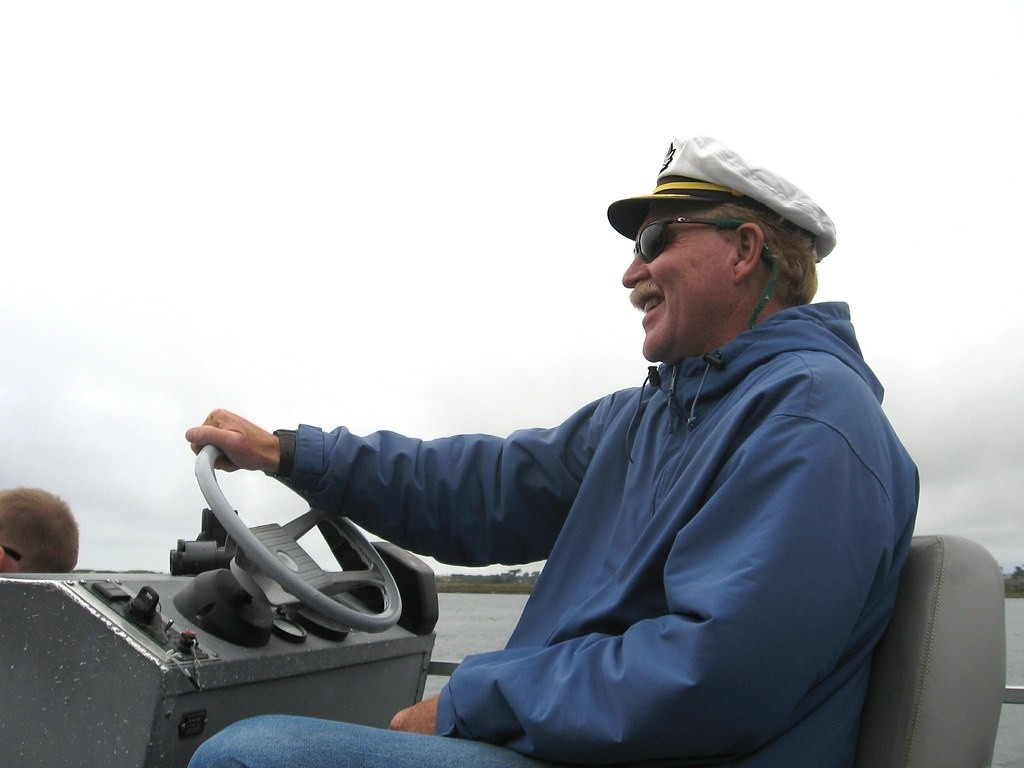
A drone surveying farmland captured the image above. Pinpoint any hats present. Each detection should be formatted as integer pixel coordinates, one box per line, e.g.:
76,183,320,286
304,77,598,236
607,135,837,263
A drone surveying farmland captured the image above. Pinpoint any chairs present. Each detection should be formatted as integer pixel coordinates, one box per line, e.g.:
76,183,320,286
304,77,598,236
854,535,1007,768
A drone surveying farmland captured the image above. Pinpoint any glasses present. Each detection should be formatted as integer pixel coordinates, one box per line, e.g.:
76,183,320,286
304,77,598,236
633,216,772,264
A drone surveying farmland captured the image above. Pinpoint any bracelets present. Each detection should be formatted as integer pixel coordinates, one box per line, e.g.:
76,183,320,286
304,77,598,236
273,429,296,479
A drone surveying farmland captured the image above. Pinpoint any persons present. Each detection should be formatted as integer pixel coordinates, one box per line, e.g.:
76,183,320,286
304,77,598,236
0,486,79,573
186,135,921,767
